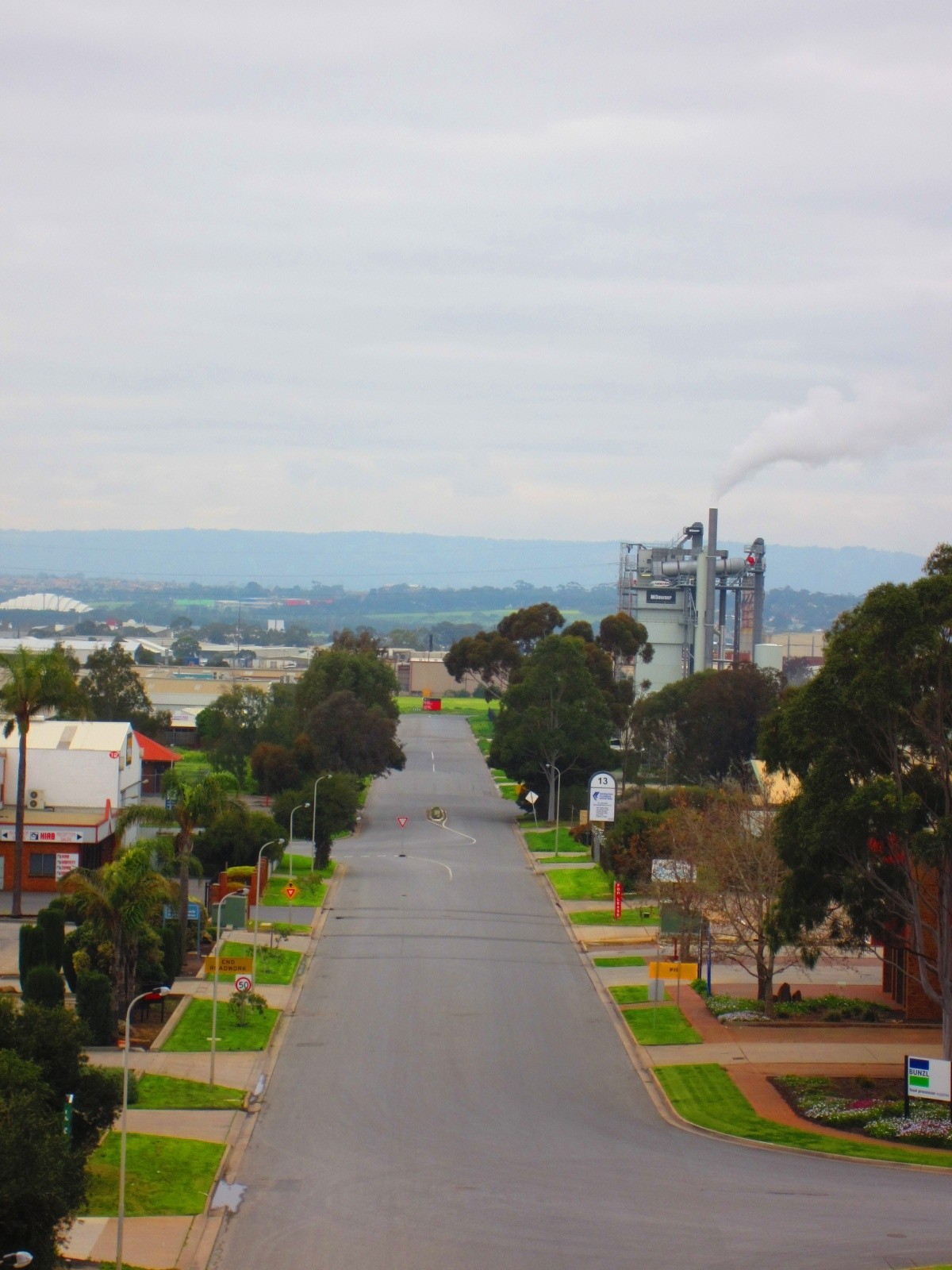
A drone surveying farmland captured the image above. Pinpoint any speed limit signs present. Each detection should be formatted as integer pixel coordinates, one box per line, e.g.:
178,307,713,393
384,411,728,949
235,977,251,992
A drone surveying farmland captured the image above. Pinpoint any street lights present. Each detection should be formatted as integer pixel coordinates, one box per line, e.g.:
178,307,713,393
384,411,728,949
288,802,310,924
208,888,251,1086
311,774,334,874
252,839,286,997
115,986,173,1269
546,764,559,858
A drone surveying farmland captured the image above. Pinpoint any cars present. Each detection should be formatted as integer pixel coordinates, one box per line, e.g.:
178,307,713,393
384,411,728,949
610,738,635,751
